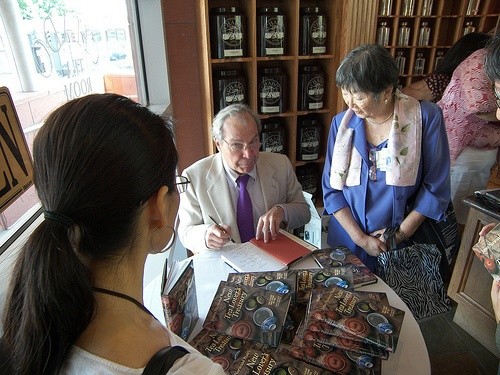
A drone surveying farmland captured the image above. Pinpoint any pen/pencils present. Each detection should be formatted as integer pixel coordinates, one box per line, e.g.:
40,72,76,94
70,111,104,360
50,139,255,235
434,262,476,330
208,215,236,244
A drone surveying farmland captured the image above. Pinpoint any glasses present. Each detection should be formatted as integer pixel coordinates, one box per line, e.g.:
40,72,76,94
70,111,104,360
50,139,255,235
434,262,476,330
222,138,262,151
172,175,191,194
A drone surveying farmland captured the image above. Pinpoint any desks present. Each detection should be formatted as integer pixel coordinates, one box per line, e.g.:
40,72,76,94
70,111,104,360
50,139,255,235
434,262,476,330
143,245,431,375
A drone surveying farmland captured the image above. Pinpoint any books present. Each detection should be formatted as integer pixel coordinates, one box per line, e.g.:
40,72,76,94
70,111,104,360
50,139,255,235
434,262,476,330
471,221,500,284
159,225,406,375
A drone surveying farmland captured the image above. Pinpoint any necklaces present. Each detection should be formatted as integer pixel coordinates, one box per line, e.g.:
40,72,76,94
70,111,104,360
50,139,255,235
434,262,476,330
93,287,159,322
366,111,394,125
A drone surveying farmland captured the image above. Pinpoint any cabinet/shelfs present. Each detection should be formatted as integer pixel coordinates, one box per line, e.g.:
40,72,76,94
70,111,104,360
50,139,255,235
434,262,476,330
197,0,500,211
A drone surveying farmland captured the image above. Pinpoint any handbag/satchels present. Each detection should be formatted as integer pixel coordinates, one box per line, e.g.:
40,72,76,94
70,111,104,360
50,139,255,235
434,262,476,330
375,224,452,321
404,191,462,281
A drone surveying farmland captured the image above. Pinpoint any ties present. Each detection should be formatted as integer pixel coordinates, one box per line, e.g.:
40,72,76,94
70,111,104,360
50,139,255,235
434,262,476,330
236,174,256,244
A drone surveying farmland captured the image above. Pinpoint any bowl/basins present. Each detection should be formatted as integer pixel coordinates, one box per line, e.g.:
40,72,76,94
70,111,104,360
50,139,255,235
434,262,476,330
167,245,397,374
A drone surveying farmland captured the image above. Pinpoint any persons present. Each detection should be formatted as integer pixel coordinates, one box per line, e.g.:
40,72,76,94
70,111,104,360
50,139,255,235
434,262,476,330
321,44,460,277
0,92,225,375
174,104,311,254
401,31,500,357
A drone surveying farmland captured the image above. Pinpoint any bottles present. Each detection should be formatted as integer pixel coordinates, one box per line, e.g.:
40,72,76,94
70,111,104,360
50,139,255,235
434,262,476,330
376,0,481,90
209,6,328,204
